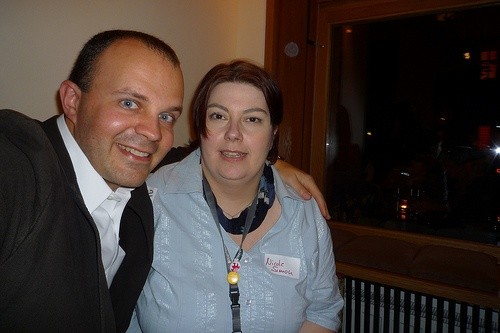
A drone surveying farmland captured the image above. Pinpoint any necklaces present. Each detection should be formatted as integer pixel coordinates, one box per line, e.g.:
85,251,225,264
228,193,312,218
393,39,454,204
200,155,276,236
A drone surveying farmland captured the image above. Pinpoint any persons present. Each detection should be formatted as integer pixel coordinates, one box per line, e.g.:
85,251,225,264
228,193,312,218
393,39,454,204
116,61,345,333
0,30,330,333
